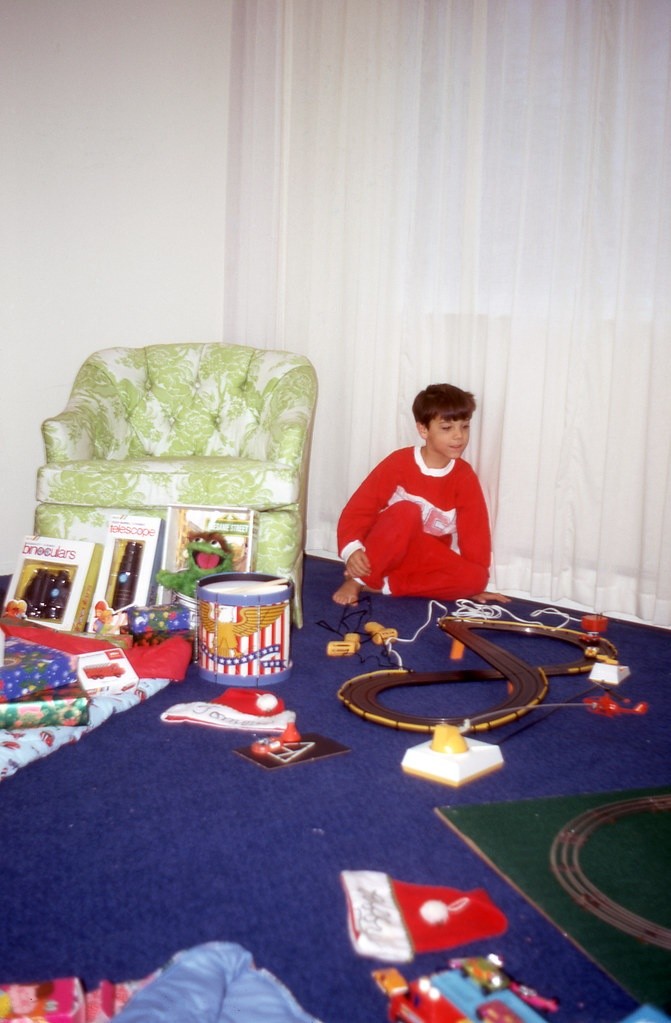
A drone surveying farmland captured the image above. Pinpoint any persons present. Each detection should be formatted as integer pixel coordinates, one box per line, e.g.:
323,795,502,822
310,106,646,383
331,384,512,606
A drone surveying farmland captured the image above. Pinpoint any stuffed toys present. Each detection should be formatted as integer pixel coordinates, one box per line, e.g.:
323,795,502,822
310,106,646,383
156,532,235,599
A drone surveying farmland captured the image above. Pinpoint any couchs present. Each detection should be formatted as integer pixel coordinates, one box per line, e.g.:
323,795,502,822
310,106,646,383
36,337,318,628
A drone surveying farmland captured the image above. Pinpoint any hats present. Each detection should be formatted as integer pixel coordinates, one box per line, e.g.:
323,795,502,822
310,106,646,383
341,869,508,963
161,688,296,733
